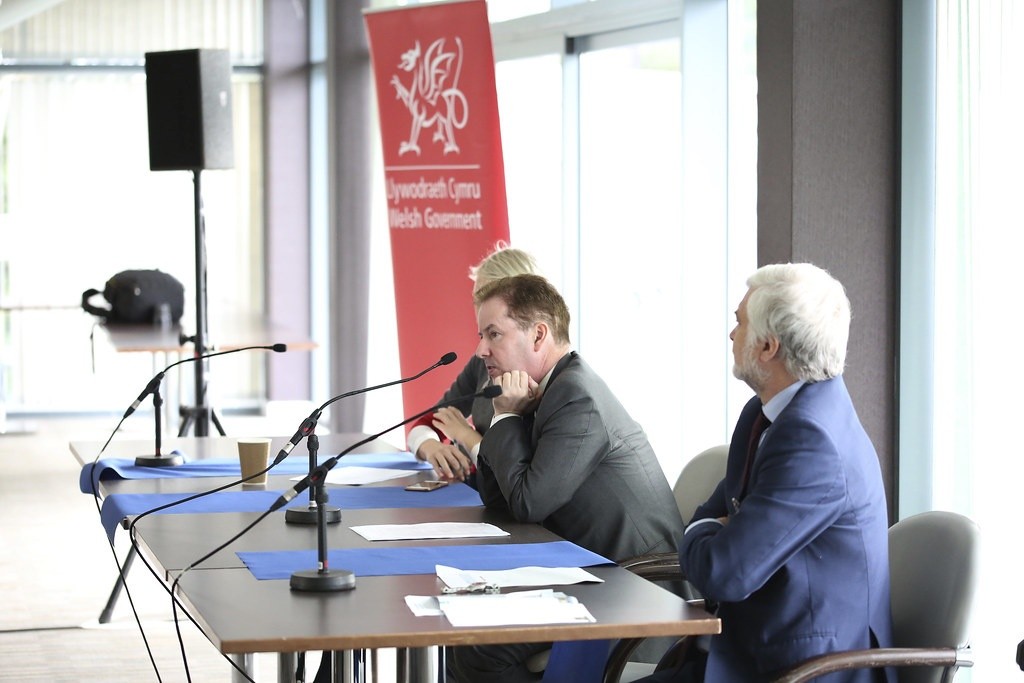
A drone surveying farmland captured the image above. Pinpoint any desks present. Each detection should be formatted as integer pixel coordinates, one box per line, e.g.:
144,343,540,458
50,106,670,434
100,318,317,431
71,432,721,683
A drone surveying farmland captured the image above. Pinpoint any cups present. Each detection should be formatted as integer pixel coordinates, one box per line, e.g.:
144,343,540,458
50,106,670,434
237,438,271,485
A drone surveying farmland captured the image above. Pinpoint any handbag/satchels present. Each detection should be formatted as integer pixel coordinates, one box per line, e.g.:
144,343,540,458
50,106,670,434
82,268,185,325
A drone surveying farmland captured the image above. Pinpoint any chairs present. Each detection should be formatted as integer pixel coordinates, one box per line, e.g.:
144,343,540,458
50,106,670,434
528,442,732,673
655,511,988,683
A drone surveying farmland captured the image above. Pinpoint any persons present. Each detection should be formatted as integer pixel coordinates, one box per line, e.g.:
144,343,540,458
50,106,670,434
310,250,542,682
447,274,687,683
678,262,904,683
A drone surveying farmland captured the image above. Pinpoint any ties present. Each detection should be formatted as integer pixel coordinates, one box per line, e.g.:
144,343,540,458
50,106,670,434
738,410,772,502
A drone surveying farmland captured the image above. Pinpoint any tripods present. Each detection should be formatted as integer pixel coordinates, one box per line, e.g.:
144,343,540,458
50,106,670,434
100,171,227,624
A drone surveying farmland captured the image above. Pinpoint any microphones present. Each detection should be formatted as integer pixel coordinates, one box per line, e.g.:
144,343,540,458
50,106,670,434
123,343,287,418
270,384,503,511
274,352,457,464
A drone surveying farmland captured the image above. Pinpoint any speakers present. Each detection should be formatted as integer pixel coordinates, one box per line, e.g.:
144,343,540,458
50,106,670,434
145,48,234,171
